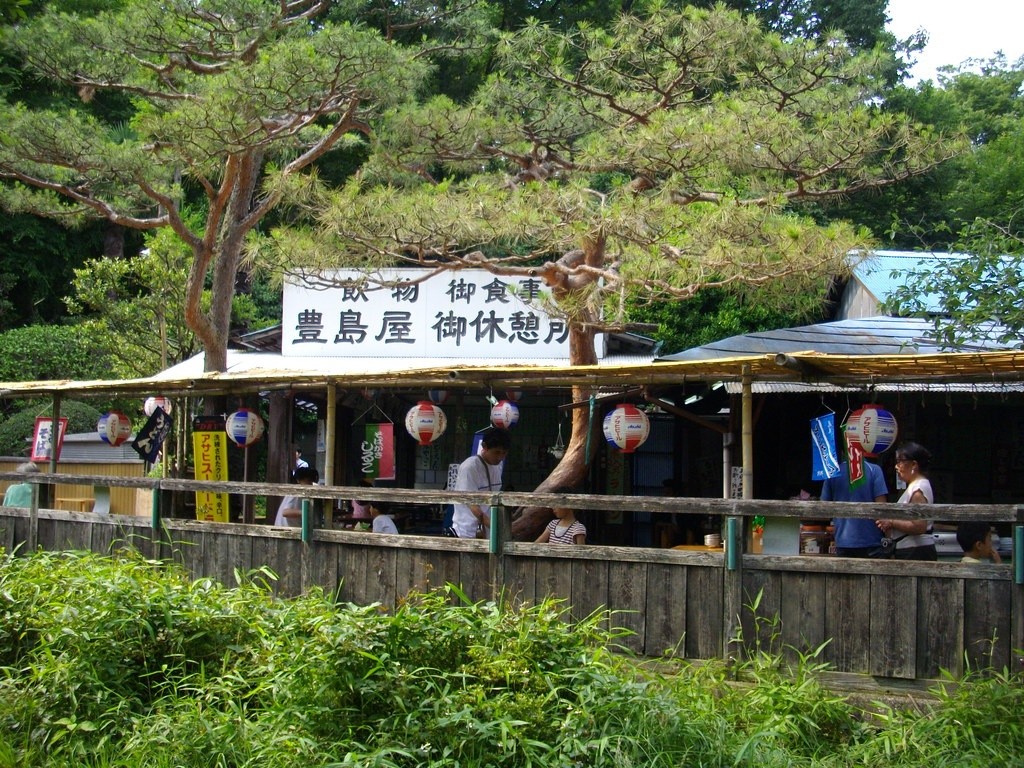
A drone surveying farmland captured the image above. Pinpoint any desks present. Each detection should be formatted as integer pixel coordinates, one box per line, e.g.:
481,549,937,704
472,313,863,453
671,544,723,553
800,531,835,554
336,512,412,534
386,504,429,527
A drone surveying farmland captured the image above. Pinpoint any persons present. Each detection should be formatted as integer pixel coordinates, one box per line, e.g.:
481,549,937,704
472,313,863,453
876,442,938,561
654,478,695,548
957,521,1002,563
451,428,509,540
819,444,888,559
3,461,41,508
274,447,399,534
535,487,587,545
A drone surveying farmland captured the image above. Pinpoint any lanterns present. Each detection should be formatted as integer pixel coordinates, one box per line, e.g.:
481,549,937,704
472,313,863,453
603,404,650,454
405,401,447,446
846,404,899,458
505,391,523,402
98,408,132,448
143,396,172,417
427,389,449,406
491,400,520,431
361,388,378,401
225,407,264,447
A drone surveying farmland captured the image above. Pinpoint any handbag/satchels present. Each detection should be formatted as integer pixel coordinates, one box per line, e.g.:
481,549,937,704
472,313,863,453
868,537,896,559
441,504,457,536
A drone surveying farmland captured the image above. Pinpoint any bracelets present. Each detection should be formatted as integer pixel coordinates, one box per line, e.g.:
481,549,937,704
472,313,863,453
889,519,893,529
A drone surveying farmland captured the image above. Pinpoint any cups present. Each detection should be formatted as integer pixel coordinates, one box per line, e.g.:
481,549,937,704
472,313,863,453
704,534,719,548
1000,538,1012,550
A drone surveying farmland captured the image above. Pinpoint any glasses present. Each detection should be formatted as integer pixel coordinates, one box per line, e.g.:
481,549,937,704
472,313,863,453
894,458,908,465
